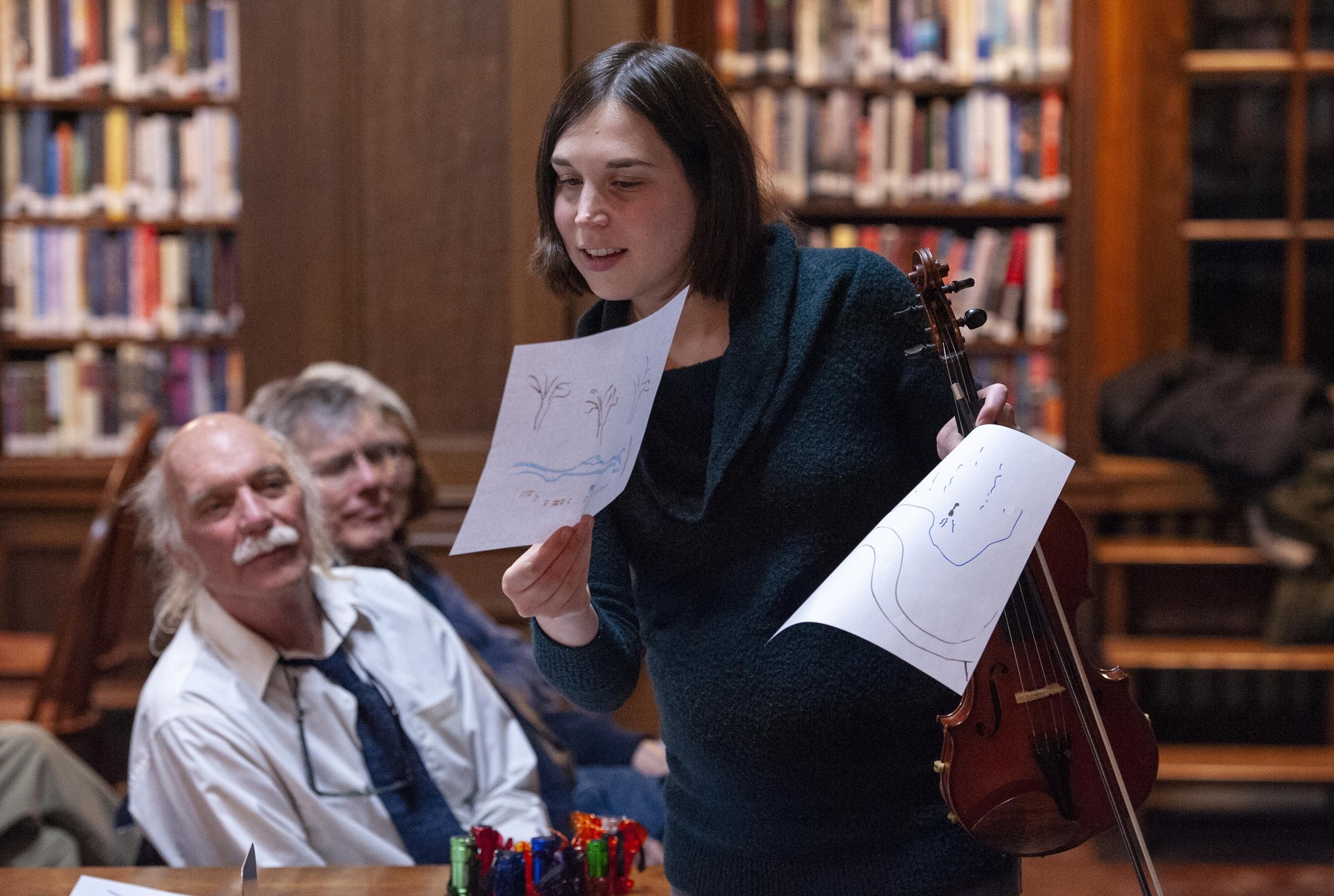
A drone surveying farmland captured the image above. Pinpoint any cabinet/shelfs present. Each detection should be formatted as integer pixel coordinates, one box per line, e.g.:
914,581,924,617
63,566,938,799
1,0,245,625
644,1,1334,846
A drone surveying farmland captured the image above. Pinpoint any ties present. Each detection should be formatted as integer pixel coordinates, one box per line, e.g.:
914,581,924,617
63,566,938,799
277,646,469,865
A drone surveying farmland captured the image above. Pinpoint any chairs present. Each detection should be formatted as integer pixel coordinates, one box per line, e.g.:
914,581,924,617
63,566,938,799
1,412,160,748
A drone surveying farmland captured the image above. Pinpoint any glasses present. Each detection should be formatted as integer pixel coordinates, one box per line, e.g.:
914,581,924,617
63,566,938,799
291,675,417,797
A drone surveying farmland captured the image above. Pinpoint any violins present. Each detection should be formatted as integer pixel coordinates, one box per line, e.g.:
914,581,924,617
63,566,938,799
890,249,1162,861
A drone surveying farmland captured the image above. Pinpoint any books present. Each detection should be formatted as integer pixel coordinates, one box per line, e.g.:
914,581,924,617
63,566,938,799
712,0,1070,455
0,0,246,460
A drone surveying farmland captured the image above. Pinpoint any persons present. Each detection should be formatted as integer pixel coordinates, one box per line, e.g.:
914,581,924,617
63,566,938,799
500,39,1027,896
0,364,672,866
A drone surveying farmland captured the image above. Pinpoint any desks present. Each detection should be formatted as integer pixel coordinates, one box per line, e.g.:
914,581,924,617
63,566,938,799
0,855,670,896
